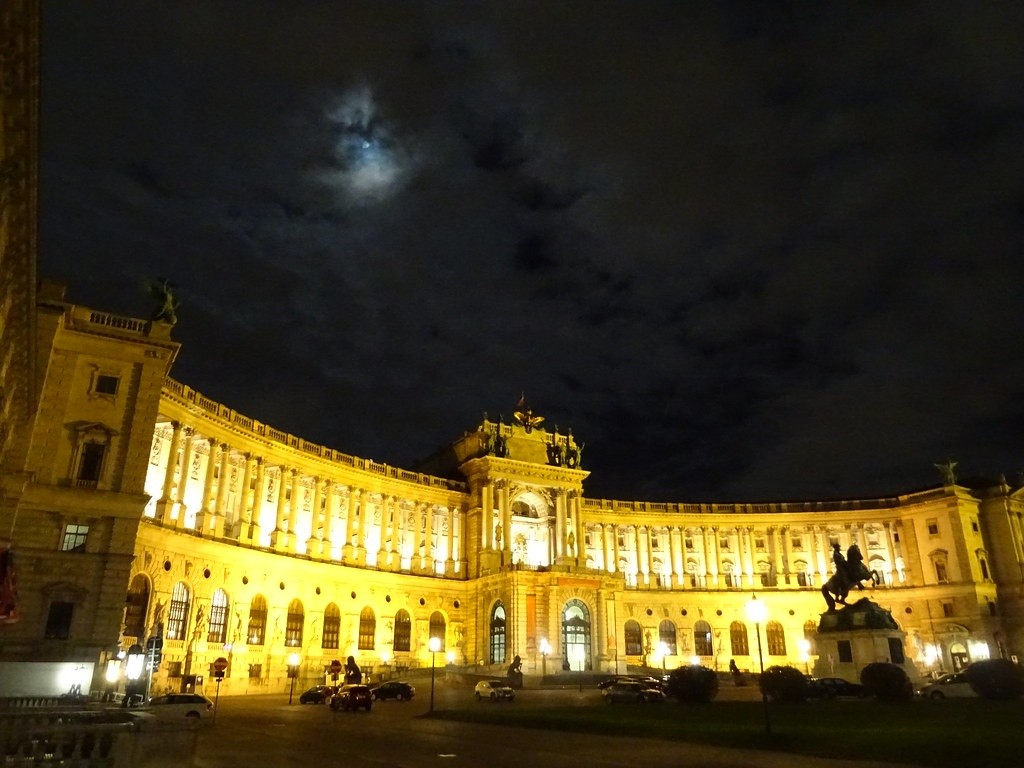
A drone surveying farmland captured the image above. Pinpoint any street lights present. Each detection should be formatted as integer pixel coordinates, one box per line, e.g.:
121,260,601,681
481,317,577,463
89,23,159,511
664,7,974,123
798,639,813,676
655,641,670,676
429,636,440,716
539,639,552,678
745,591,773,750
288,654,300,706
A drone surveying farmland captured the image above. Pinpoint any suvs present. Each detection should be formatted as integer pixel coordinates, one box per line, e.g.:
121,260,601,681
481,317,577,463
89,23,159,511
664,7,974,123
370,681,415,701
637,677,663,689
475,680,516,702
329,683,372,710
131,694,214,729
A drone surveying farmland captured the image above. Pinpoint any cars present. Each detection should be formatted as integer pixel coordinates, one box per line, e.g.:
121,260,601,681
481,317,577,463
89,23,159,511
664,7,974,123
598,676,636,689
601,681,666,706
299,686,331,704
916,671,977,699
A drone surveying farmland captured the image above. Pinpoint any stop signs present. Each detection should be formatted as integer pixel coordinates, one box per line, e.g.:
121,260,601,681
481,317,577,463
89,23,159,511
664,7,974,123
327,662,341,674
214,657,228,670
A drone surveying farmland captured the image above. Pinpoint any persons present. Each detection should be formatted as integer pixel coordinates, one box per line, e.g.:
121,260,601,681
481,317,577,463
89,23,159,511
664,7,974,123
833,543,844,569
